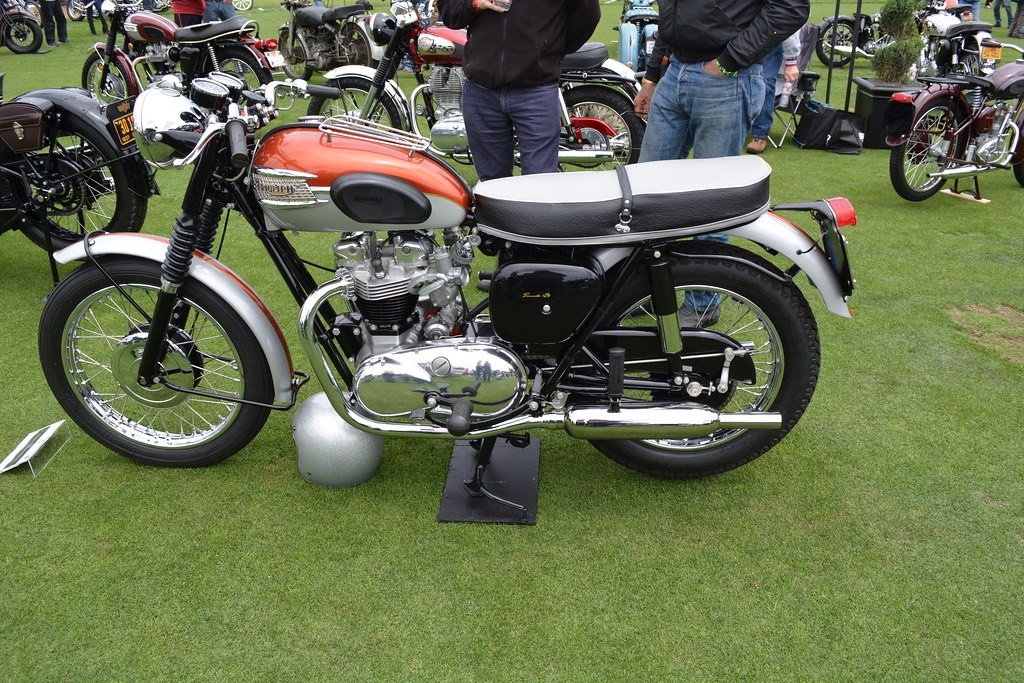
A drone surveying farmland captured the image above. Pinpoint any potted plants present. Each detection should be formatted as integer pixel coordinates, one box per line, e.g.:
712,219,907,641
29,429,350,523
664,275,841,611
852,0,929,148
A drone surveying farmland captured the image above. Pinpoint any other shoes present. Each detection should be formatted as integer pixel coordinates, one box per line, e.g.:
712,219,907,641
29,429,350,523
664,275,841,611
1008,25,1011,28
623,298,653,318
746,138,769,153
677,303,721,330
992,22,1001,27
985,2,993,9
103,28,109,33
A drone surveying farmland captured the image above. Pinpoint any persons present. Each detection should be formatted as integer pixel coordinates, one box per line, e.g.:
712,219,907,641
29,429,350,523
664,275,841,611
993,0,1013,27
747,30,801,154
435,0,601,182
985,0,993,8
84,0,110,35
39,0,69,47
203,0,236,23
958,0,981,21
631,0,811,327
170,0,206,27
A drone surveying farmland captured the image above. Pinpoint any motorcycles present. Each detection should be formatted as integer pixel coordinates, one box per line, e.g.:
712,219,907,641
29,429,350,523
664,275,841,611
0,0,256,54
0,72,164,255
34,68,858,527
607,0,658,72
885,39,1023,202
817,0,999,79
278,0,397,81
73,0,280,113
308,0,647,173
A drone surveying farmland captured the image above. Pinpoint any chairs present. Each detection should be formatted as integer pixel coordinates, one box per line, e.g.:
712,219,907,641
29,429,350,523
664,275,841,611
767,24,821,148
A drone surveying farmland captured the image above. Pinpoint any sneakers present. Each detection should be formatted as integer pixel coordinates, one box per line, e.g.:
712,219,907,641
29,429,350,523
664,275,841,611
46,42,58,47
59,40,69,43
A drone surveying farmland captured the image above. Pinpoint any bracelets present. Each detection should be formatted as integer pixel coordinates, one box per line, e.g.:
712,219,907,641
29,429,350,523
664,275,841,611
474,0,480,11
642,77,653,84
717,60,734,77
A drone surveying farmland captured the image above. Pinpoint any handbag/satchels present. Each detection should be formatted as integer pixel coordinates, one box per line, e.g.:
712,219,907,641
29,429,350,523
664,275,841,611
793,99,866,155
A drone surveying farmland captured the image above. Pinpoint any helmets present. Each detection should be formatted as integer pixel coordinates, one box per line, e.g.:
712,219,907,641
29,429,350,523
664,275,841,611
291,391,384,487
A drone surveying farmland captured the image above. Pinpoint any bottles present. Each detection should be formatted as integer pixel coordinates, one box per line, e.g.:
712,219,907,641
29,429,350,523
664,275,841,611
493,0,513,11
779,81,792,107
